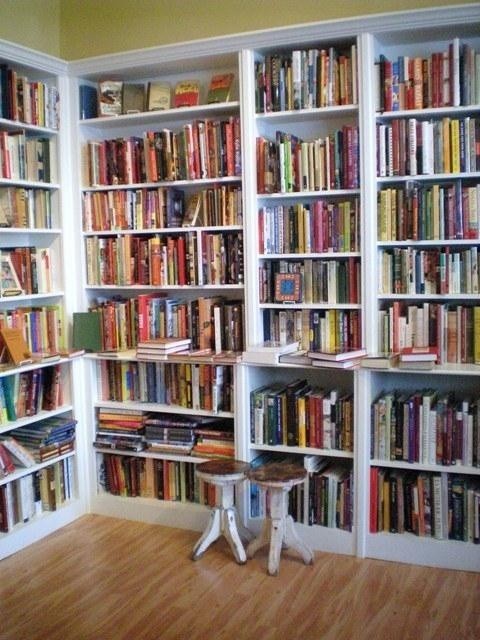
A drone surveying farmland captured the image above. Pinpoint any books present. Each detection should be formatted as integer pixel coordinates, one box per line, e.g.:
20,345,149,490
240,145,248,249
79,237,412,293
254,43,359,531
78,74,246,507
368,36,480,543
2,62,77,534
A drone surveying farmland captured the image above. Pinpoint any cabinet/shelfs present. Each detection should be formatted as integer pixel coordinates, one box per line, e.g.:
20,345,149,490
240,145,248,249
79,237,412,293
0,39,83,568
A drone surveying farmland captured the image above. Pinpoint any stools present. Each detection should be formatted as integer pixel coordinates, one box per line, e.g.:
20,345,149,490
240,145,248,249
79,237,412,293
190,456,253,563
247,462,316,577
